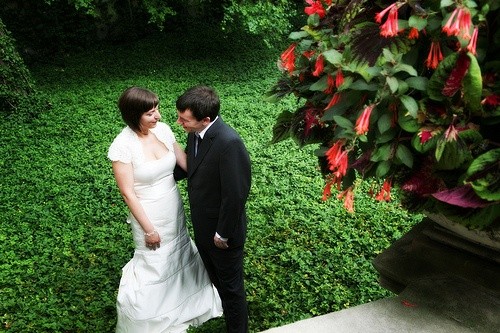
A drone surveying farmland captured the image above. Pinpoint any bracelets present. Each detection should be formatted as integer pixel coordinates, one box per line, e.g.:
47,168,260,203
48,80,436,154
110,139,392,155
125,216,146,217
145,229,158,236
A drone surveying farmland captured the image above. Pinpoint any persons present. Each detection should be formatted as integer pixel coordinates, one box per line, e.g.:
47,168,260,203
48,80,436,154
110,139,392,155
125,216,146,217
174,86,251,333
106,86,223,333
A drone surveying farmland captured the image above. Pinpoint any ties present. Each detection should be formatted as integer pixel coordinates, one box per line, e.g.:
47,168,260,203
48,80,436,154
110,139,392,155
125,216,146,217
194,132,199,158
